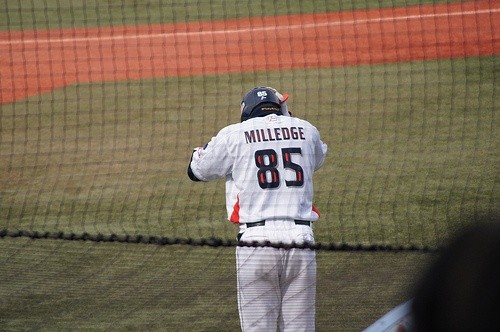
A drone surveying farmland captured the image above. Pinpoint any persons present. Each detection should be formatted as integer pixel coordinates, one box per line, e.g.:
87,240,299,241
187,87,327,332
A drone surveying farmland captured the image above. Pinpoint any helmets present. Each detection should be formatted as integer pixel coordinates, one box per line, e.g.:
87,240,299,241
240,87,290,122
